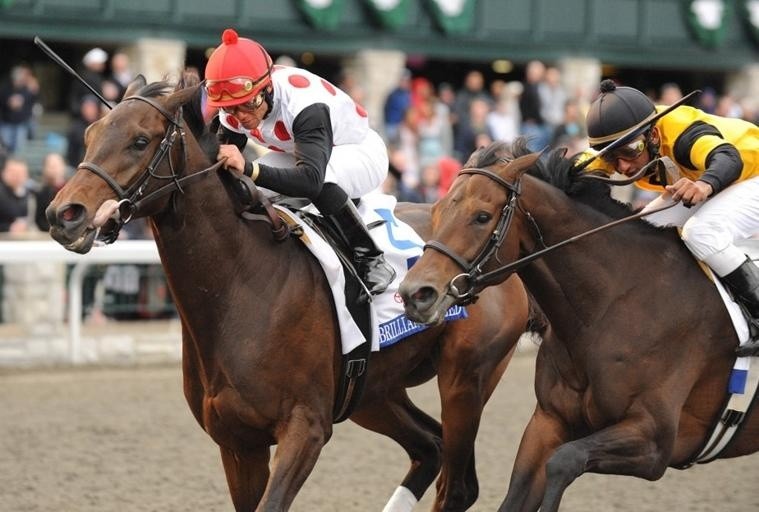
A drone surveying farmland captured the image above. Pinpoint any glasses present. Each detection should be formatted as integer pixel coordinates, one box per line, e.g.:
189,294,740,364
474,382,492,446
222,88,266,115
600,138,650,162
206,76,253,101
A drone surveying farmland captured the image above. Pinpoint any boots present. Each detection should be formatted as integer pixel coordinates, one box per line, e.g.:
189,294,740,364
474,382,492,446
322,197,395,304
720,253,759,356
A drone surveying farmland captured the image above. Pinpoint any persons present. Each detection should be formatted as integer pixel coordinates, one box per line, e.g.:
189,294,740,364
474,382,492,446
1,49,759,233
573,78,759,357
205,28,396,306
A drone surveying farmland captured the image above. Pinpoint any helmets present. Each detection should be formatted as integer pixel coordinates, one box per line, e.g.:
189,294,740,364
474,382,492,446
585,79,657,150
205,28,274,107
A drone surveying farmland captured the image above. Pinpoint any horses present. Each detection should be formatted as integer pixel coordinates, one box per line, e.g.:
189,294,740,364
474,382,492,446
45,67,549,512
397,134,759,511
